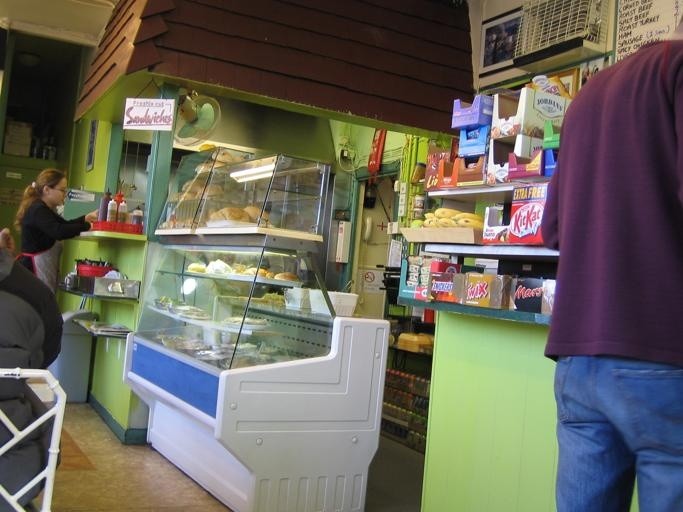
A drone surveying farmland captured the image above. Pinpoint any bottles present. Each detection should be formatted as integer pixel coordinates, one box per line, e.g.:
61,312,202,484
409,161,427,184
379,366,429,450
97,187,143,225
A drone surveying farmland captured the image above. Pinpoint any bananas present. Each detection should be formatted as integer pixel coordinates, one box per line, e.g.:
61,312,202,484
410,208,484,230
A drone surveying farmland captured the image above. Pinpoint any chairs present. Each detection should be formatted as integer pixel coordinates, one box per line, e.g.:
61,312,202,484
0,367,68,512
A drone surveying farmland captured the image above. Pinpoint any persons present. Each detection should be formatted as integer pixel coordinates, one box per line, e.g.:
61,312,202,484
17,168,100,298
582,60,599,83
539,13,683,512
0,228,61,512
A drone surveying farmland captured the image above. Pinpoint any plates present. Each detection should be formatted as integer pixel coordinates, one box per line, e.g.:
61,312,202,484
169,309,210,319
225,322,271,330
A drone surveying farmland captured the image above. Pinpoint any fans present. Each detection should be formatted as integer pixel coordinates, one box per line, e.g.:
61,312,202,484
173,93,222,148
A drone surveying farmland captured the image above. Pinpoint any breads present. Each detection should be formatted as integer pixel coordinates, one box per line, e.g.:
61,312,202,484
274,272,299,281
212,205,269,224
181,150,244,200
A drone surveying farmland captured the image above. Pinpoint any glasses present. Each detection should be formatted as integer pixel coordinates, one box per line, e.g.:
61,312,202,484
42,185,68,194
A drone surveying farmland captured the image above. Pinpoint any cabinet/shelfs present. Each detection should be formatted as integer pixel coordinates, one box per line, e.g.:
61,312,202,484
58,229,147,340
395,175,559,325
133,147,253,231
123,231,393,512
198,152,333,236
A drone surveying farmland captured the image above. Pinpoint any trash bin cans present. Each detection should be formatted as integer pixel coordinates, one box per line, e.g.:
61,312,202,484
46,311,97,404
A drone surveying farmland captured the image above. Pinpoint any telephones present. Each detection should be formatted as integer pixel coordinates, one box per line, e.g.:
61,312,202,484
362,217,372,241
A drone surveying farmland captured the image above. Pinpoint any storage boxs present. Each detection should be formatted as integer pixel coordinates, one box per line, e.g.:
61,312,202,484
420,85,571,192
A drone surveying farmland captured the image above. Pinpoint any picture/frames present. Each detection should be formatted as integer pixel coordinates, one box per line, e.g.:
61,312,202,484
478,4,522,78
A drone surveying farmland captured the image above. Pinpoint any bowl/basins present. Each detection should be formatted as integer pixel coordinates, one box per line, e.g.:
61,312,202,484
76,264,112,277
89,220,142,234
31,147,49,160
307,288,359,317
